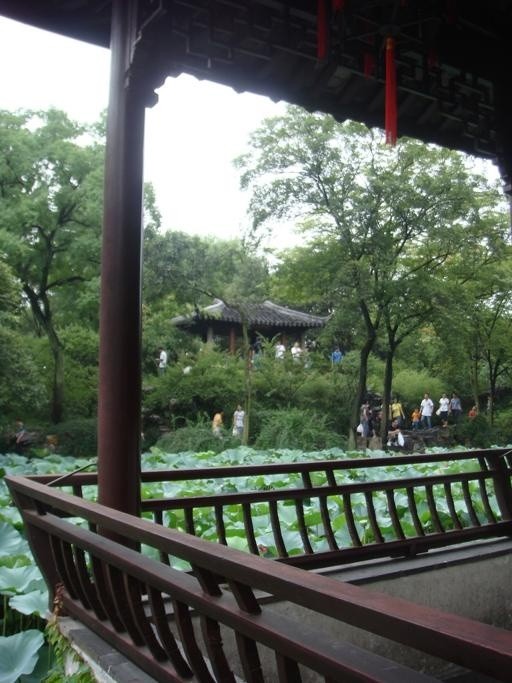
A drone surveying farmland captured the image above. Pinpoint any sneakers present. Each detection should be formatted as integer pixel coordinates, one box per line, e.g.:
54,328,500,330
393,442,398,447
387,442,391,446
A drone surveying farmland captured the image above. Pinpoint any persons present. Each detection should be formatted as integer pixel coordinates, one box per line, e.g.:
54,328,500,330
156,346,167,376
212,408,224,440
357,390,479,451
231,404,246,439
274,338,313,368
332,346,342,370
